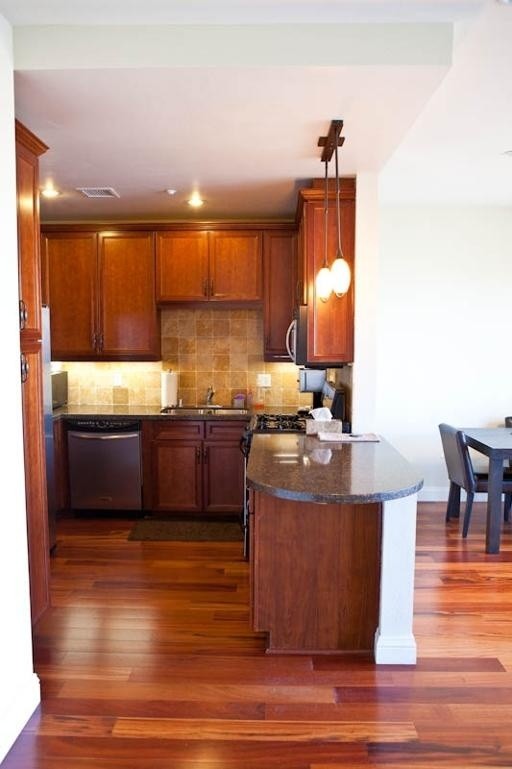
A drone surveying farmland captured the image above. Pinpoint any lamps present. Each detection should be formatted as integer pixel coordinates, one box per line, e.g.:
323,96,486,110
317,119,350,309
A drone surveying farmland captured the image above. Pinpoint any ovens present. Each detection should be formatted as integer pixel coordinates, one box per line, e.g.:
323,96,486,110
66,430,142,513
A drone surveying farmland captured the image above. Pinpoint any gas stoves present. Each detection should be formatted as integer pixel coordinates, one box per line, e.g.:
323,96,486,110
250,413,313,433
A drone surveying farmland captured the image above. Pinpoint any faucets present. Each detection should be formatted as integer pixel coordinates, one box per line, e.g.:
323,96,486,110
203,385,217,406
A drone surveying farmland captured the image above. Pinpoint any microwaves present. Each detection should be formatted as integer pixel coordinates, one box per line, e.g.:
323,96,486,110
284,306,342,368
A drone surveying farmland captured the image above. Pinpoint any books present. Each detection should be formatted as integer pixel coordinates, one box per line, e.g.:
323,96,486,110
317,432,380,442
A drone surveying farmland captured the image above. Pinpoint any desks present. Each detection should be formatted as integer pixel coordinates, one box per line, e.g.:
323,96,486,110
461,424,511,554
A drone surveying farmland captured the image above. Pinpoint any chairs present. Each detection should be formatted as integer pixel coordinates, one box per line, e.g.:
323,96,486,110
435,422,512,538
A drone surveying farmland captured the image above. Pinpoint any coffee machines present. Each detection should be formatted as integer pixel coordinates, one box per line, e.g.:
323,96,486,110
295,368,329,417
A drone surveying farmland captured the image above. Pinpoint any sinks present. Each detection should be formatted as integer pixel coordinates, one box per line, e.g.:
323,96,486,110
207,409,249,416
159,407,208,415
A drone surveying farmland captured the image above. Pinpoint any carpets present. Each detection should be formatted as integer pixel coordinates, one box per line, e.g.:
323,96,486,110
127,518,243,543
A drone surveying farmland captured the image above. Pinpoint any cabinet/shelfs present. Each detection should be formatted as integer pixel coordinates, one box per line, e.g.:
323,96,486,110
17,120,52,341
156,226,264,308
21,342,55,626
293,177,353,368
242,484,387,660
142,422,243,513
264,222,291,362
41,223,161,361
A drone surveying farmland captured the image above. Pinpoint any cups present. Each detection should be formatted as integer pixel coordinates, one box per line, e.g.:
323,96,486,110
233,398,245,408
253,398,265,411
